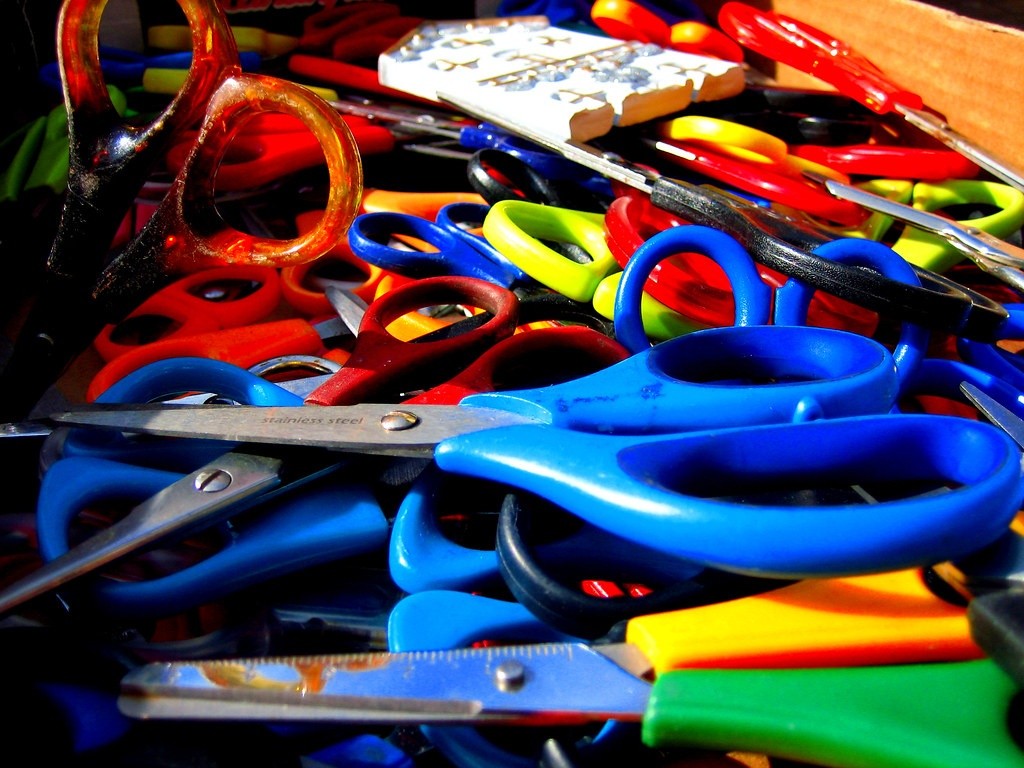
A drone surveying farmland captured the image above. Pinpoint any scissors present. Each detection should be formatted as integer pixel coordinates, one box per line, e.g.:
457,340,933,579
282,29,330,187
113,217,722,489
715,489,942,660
0,0,1024,768
